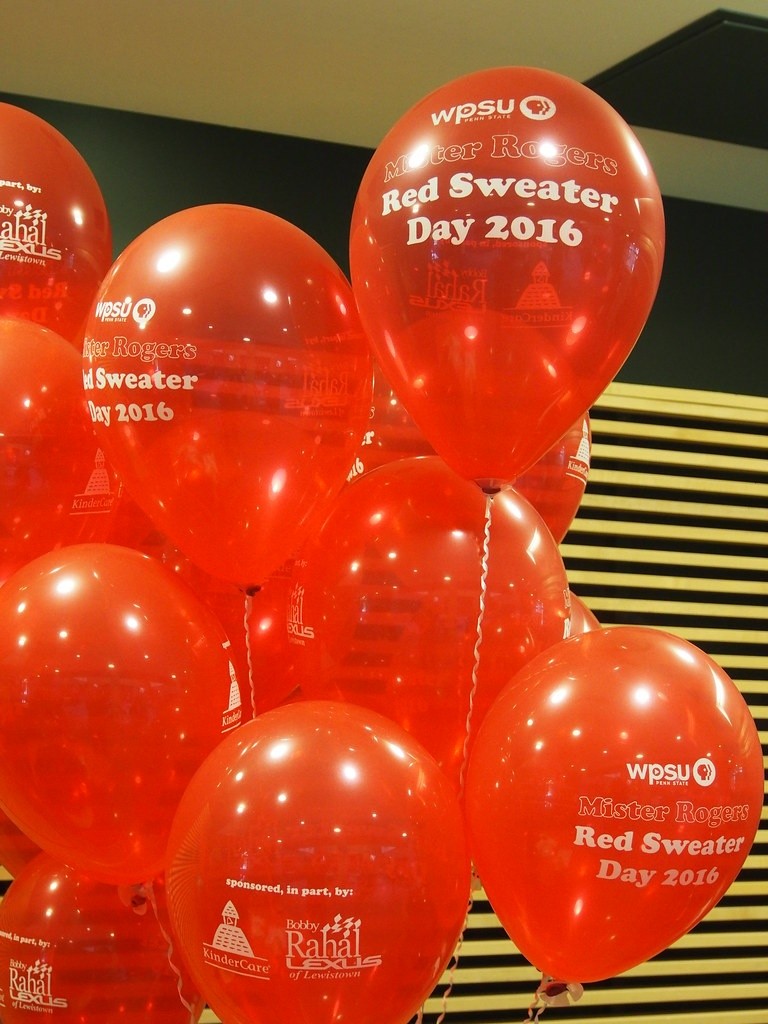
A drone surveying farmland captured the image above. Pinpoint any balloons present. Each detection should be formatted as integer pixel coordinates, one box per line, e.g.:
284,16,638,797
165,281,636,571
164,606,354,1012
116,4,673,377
0,321,122,582
0,104,112,355
0,810,207,1024
110,493,298,718
83,204,366,595
0,544,238,883
368,307,601,635
285,454,569,763
464,629,762,994
165,672,472,1024
349,68,666,495
